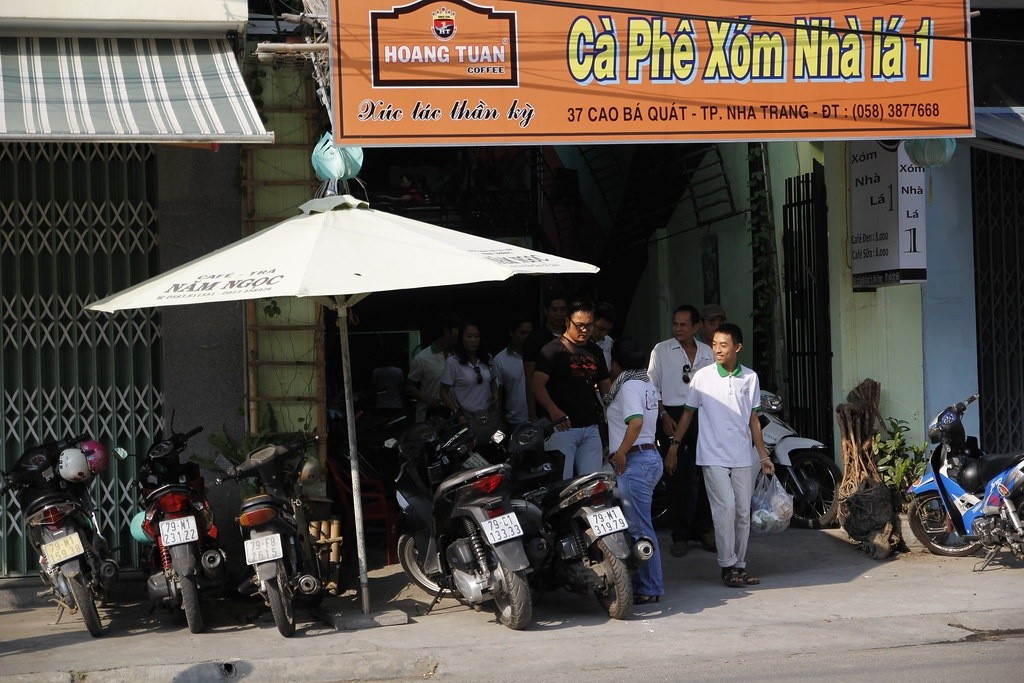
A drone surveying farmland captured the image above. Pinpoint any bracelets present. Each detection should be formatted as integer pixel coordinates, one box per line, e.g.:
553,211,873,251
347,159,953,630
760,457,770,462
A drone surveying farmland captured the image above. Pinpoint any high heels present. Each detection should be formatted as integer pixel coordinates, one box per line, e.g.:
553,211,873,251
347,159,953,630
634,592,660,605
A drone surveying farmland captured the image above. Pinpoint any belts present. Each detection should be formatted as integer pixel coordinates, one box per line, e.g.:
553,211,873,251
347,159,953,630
611,444,655,457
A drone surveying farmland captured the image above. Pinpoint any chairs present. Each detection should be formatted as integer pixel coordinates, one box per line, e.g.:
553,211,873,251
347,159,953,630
325,457,397,566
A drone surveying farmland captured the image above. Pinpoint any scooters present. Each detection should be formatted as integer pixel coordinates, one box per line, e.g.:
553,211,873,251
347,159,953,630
0,431,126,639
215,429,326,639
111,407,229,636
380,392,843,631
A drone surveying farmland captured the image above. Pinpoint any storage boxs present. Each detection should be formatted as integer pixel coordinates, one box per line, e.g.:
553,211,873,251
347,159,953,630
305,496,333,522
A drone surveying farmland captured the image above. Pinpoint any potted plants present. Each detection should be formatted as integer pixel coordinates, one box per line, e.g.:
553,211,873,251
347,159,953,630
870,416,928,553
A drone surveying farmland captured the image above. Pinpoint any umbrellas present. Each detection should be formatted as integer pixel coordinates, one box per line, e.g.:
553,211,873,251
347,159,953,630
83,191,600,615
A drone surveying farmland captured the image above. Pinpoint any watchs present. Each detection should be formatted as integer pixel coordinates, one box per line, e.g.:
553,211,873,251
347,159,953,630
670,439,681,445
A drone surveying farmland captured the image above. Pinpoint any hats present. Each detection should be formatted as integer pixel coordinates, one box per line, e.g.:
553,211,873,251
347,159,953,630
699,304,727,322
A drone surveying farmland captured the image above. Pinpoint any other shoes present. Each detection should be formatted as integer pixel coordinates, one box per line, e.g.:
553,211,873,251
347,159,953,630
671,542,687,556
700,532,717,551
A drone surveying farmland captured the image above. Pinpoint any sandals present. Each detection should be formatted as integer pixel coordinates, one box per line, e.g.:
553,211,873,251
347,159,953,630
721,565,747,586
740,568,761,585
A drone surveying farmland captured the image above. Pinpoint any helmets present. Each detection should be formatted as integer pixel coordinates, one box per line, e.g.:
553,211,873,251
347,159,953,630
60,449,91,483
297,453,321,486
80,441,108,473
957,459,984,494
130,510,155,544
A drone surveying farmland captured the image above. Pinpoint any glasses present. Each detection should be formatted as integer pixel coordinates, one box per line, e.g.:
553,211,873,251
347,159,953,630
682,364,690,383
474,366,483,384
569,317,594,333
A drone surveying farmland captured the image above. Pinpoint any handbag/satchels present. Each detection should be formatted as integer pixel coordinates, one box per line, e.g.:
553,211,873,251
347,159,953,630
752,473,793,533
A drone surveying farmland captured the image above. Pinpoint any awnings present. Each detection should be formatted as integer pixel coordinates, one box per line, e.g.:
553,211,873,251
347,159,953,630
956,112,1024,159
0,32,274,144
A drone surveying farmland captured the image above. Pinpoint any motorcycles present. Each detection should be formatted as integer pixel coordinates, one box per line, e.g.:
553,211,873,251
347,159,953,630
902,392,1024,573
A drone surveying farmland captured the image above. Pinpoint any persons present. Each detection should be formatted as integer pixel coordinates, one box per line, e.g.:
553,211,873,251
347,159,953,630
523,293,615,480
439,323,508,464
490,317,533,430
378,172,427,206
368,349,405,418
644,304,727,557
406,317,465,424
606,335,665,604
665,323,775,586
